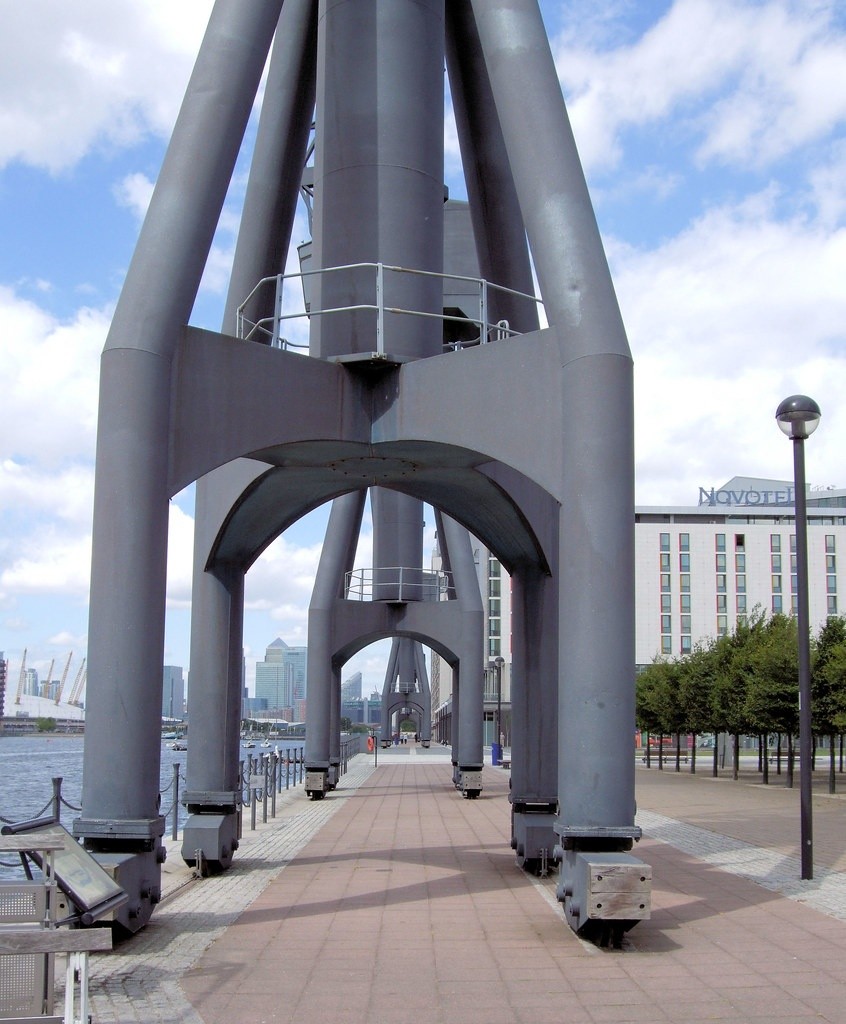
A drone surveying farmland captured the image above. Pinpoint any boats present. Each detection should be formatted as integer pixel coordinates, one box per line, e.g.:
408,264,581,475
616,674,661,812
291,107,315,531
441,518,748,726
243,740,257,749
259,737,278,748
168,739,188,752
161,728,185,740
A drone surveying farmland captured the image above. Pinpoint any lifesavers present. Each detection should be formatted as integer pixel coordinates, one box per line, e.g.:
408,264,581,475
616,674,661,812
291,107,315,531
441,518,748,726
367,737,374,751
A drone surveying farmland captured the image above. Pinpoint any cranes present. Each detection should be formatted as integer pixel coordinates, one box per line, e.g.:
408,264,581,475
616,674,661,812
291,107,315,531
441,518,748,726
15,647,28,705
55,650,73,705
74,669,87,706
67,657,87,705
43,659,56,699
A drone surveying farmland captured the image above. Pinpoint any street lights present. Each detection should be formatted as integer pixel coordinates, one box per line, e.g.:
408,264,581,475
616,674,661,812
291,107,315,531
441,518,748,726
434,702,449,747
774,395,837,880
494,655,505,767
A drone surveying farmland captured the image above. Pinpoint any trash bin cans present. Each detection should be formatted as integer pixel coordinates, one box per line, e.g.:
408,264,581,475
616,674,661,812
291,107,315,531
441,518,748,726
491,742,503,766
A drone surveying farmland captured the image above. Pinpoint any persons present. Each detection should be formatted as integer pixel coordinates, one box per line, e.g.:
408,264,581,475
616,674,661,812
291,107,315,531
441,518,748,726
394,734,399,747
400,733,404,744
414,733,418,742
441,738,449,745
404,733,407,744
500,732,505,748
